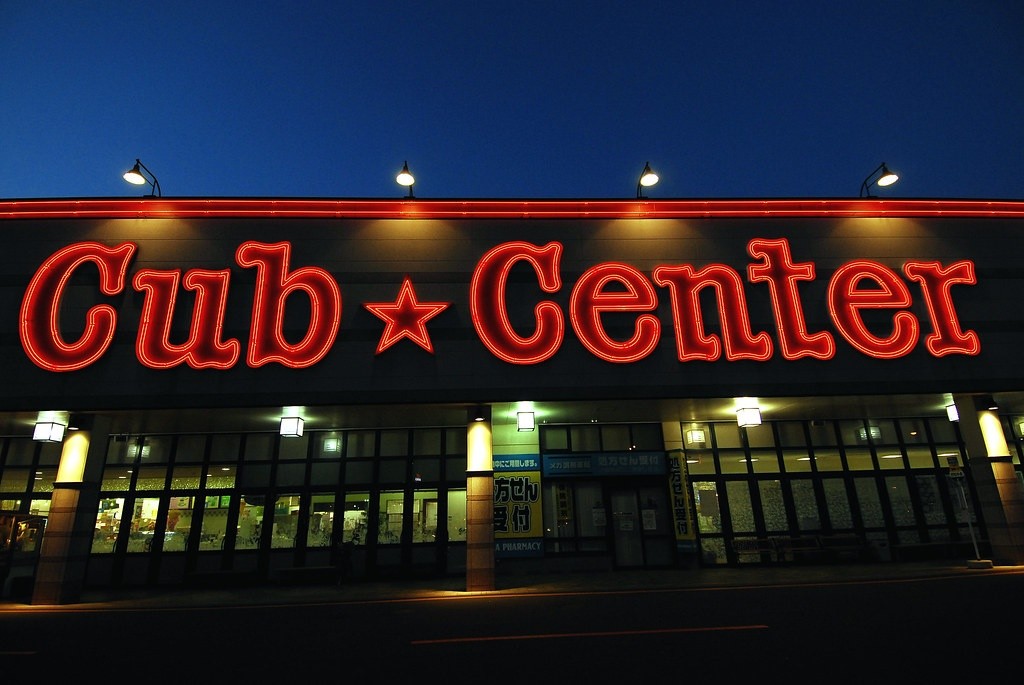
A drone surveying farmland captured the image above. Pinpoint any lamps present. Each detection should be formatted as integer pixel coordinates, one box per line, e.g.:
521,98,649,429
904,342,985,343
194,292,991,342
517,412,535,431
860,162,899,198
736,408,762,428
127,440,151,458
33,422,66,443
686,423,706,444
856,426,882,440
395,161,417,199
637,161,659,199
280,416,305,438
324,432,342,452
123,158,162,199
945,404,960,422
